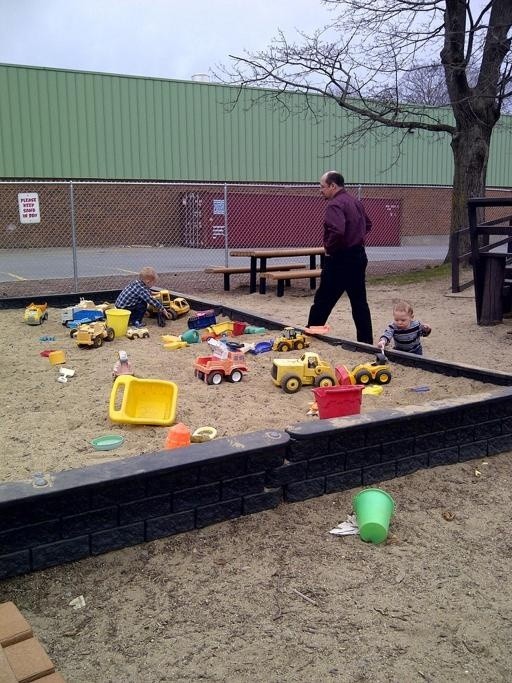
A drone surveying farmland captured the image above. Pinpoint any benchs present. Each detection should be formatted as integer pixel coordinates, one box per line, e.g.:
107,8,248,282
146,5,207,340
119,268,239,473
259,268,323,297
205,263,307,291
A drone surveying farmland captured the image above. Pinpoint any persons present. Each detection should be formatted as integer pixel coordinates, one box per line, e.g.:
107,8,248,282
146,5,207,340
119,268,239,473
377,301,431,355
117,266,173,324
308,170,374,346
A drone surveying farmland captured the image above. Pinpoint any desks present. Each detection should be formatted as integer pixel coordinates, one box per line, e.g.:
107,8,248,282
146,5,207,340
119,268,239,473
230,246,326,294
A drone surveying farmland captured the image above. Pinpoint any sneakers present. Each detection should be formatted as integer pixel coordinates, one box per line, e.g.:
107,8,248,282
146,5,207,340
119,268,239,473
130,320,146,327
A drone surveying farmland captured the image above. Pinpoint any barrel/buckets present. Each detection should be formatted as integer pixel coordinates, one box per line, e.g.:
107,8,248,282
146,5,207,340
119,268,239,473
105,308,131,336
311,384,365,419
352,488,395,544
